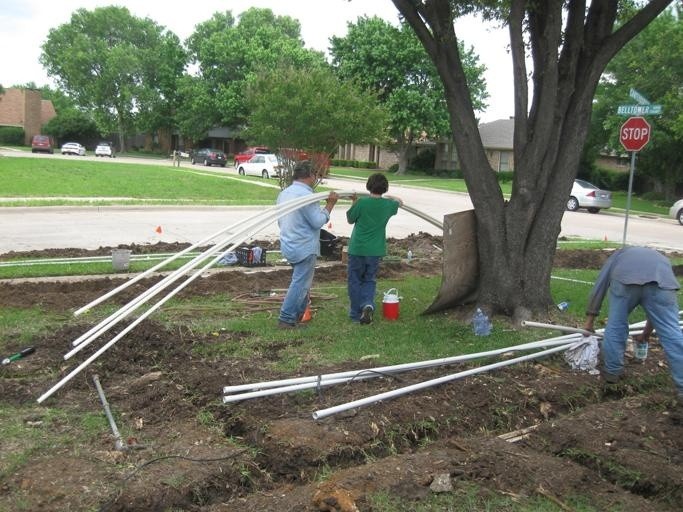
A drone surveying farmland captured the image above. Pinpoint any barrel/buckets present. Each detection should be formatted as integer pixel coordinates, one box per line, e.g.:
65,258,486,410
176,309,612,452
471,307,490,336
382,287,400,321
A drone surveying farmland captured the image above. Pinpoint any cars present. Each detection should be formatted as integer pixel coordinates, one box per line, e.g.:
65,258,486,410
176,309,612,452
562,178,612,216
61,141,88,156
667,199,683,227
93,141,116,157
175,142,332,181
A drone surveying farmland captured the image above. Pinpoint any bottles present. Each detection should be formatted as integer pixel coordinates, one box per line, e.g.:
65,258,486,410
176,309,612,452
408,251,412,260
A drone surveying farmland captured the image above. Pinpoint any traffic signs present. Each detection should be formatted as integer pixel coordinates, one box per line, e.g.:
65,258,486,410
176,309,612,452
628,88,653,106
618,103,662,116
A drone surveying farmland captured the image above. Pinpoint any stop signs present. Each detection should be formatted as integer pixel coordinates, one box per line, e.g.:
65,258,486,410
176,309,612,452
618,116,653,151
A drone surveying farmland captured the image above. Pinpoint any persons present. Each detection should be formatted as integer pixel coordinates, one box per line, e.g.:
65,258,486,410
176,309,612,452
579,245,682,404
345,171,403,326
274,159,340,331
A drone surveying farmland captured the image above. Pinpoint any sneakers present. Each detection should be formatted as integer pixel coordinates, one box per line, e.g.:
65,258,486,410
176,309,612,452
600,367,619,384
360,304,373,324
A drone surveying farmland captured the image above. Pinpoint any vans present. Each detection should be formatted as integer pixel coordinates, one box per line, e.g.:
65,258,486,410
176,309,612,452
31,134,55,154
